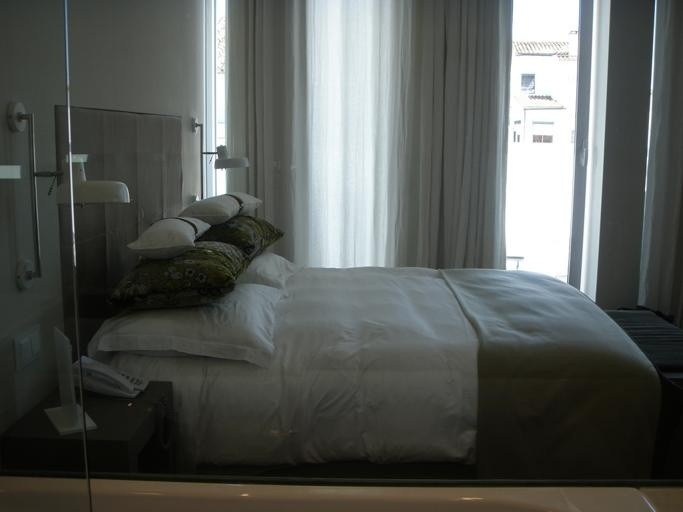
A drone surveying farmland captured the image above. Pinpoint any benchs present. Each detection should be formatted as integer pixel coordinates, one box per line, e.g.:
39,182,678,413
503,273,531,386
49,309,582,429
603,307,682,421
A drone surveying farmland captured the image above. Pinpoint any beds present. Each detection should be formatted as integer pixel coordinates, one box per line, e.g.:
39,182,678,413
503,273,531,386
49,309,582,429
72,265,663,487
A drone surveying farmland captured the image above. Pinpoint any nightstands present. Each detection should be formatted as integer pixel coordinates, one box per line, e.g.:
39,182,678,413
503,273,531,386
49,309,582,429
0,380,174,480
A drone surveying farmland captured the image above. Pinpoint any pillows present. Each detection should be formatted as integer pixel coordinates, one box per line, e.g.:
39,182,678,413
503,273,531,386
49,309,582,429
84,192,301,370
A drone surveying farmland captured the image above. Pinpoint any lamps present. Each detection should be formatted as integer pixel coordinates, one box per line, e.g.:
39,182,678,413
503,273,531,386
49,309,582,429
189,114,250,206
6,99,132,294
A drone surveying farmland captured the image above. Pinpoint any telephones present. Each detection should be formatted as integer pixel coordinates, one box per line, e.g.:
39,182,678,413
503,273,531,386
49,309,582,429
72,355,150,398
